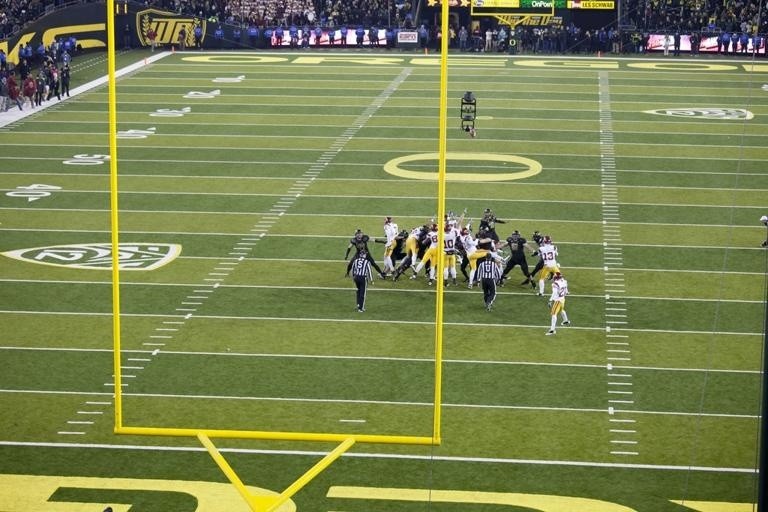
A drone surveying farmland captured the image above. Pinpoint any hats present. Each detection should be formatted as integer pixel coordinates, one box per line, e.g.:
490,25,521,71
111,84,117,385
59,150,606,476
359,250,368,254
487,253,491,258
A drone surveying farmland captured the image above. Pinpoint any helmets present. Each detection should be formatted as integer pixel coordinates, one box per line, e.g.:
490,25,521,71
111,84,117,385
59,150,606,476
355,229,364,238
399,231,409,239
444,211,469,235
410,222,438,233
512,230,521,238
552,273,562,280
533,230,540,236
544,236,552,245
385,216,393,224
478,208,493,238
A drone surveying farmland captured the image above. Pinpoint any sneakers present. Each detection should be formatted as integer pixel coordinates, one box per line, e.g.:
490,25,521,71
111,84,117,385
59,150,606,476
410,264,415,272
496,276,511,287
358,308,366,313
485,303,492,311
561,320,571,326
444,271,459,288
378,270,401,283
413,274,416,279
467,276,482,289
545,329,557,336
424,269,437,286
521,278,544,297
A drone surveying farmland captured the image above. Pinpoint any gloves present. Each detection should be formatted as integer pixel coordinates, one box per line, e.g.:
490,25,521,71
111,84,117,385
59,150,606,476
534,251,539,256
547,302,553,309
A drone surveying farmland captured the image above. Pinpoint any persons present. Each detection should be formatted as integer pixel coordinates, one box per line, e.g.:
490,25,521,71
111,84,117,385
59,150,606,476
344,210,570,334
450,0,768,56
759,216,768,247
142,0,440,54
0,0,77,110
